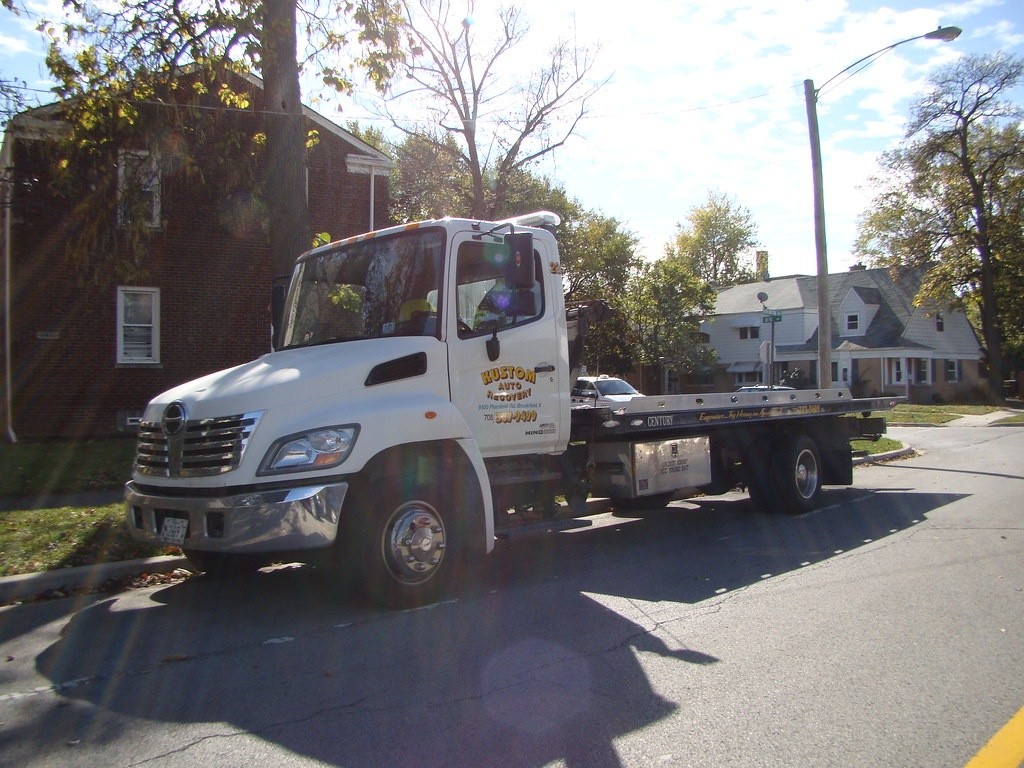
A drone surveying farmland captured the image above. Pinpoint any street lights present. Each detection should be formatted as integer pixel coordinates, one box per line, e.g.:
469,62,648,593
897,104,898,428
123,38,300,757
803,24,965,412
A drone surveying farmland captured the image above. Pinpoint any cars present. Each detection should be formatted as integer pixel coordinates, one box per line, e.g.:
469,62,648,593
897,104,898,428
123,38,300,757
736,386,795,393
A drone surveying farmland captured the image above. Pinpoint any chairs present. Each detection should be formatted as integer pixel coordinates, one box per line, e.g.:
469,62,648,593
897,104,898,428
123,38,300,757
394,292,533,332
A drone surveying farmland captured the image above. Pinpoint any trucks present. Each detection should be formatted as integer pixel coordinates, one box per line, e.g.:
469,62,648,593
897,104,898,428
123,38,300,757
124,210,906,615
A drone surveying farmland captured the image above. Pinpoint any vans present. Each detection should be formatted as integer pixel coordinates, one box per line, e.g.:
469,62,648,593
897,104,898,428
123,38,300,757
569,374,648,412
